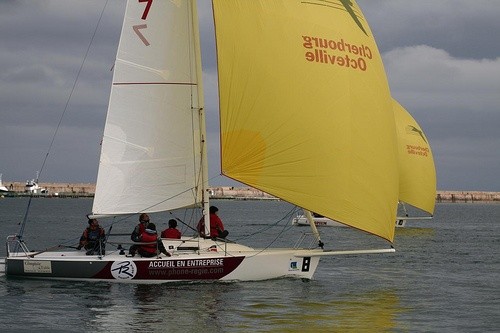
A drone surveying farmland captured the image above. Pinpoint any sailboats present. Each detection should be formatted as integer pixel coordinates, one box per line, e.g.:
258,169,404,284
4,1,438,283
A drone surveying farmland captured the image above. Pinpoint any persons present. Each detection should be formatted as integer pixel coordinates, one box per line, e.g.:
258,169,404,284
196,206,229,241
161,219,181,240
129,213,171,258
78,219,106,255
